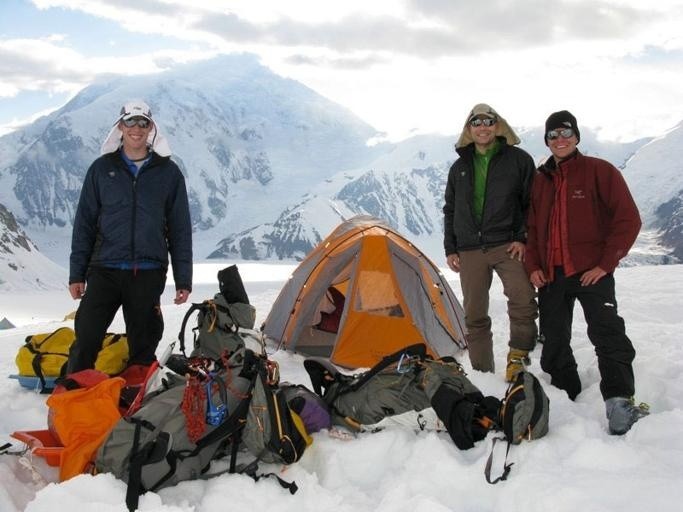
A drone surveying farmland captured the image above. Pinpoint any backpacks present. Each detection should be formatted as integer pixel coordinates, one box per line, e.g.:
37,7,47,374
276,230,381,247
9,293,331,512
506,372,550,445
325,354,501,450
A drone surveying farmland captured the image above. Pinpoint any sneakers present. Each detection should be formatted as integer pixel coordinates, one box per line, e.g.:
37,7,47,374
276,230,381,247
606,397,650,435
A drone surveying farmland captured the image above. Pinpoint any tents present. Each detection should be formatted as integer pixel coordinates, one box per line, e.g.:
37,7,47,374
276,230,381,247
262,216,468,360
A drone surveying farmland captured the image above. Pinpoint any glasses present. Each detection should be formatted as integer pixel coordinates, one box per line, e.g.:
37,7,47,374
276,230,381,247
469,119,495,126
547,129,573,139
124,117,149,128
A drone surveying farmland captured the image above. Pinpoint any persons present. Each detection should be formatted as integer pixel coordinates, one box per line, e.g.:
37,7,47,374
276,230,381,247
66,100,192,377
306,350,548,451
442,104,534,383
528,109,651,433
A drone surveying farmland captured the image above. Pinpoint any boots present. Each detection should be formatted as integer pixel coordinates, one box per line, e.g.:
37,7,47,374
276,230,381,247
505,349,532,383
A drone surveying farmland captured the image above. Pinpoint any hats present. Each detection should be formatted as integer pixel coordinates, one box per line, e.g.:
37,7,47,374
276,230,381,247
544,111,580,146
